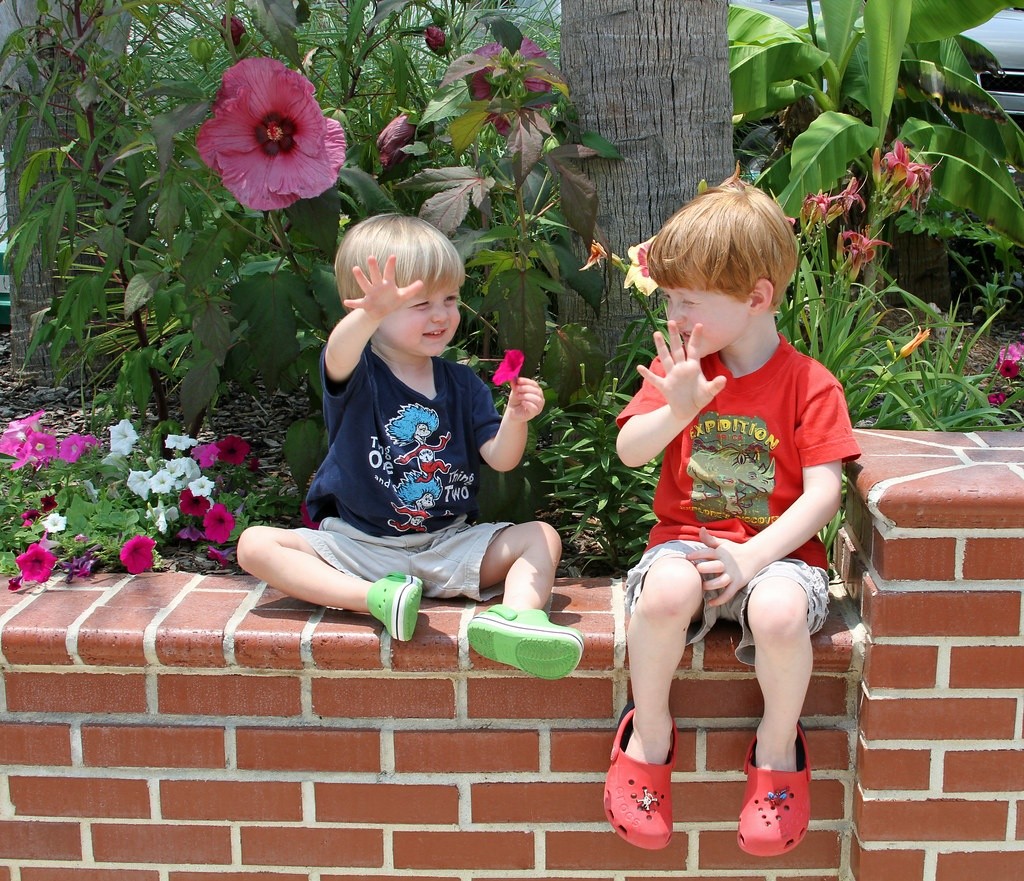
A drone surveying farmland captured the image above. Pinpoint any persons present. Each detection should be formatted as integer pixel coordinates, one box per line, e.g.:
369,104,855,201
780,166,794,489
604,185,861,857
237,214,585,680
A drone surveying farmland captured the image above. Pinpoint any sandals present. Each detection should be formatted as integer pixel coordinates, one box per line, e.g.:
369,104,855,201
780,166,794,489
602,702,677,849
737,717,812,857
467,604,585,679
365,572,424,641
